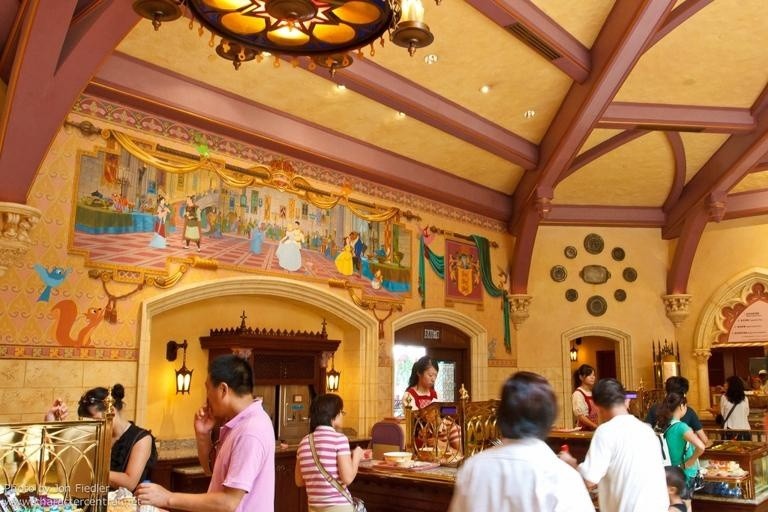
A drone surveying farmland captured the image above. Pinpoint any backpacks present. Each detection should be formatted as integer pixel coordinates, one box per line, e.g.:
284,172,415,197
657,421,690,466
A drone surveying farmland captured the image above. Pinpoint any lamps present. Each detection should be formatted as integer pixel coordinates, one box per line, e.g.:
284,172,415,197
570,344,578,362
131,0,441,77
326,353,340,392
175,339,194,394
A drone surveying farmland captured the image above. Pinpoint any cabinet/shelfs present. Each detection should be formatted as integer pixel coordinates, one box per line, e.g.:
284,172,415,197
149,438,372,512
198,310,341,438
348,456,599,512
546,429,768,512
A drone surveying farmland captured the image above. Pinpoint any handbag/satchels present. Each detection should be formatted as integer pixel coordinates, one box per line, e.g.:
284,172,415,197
352,498,367,512
715,414,725,426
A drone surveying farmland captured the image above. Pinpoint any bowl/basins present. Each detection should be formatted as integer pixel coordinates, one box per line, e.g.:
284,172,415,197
418,447,440,461
383,452,413,468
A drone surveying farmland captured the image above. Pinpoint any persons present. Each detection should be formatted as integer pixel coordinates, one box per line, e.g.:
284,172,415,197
111,194,364,279
447,370,596,512
44,398,69,424
132,354,277,512
294,393,365,511
644,376,710,491
758,369,768,392
655,392,706,512
663,465,688,512
720,375,752,440
45,383,157,495
571,364,598,430
402,356,439,410
561,377,671,512
418,409,462,464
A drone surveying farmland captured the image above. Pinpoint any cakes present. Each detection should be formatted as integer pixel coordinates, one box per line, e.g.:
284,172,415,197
703,460,749,478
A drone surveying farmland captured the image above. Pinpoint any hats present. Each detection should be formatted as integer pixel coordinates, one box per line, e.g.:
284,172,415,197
592,379,638,403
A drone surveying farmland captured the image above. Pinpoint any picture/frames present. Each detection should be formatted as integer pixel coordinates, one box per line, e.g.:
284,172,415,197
443,237,485,312
65,140,419,312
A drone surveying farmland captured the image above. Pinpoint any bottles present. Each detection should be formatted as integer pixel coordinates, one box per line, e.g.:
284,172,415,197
140,480,153,512
